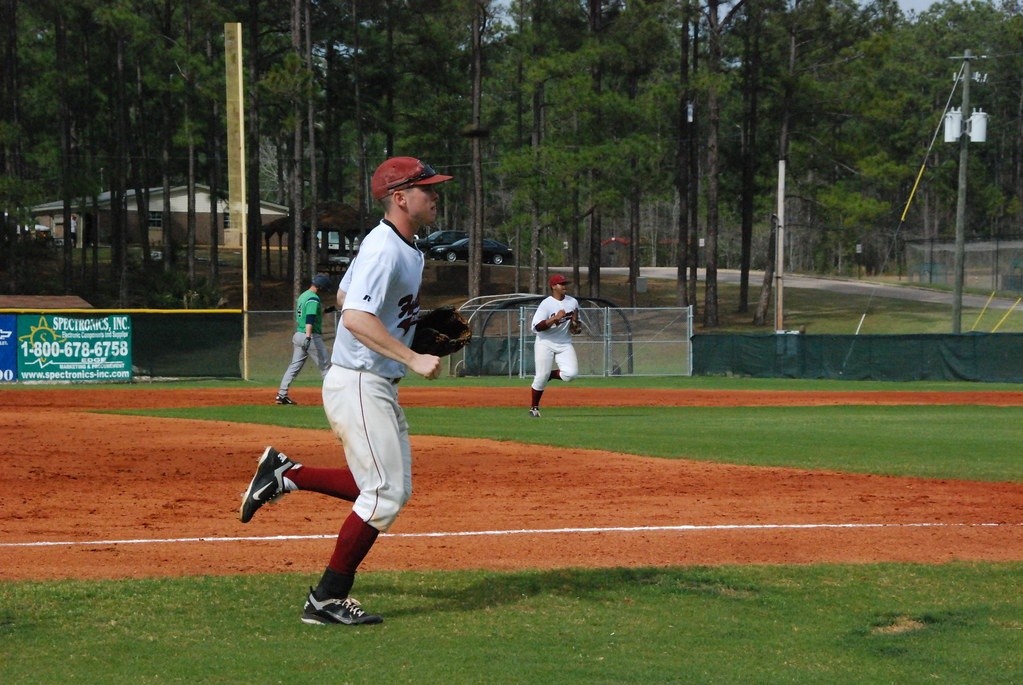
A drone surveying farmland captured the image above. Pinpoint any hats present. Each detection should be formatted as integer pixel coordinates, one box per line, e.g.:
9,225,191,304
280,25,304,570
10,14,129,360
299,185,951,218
549,275,571,287
371,157,454,201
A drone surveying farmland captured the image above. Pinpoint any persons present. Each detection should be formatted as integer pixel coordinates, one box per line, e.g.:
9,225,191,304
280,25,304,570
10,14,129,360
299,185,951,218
237,156,472,626
274,274,333,406
71,215,77,247
530,275,582,417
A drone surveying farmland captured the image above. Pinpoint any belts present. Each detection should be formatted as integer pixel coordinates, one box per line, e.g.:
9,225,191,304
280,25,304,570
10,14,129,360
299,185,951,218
387,377,401,383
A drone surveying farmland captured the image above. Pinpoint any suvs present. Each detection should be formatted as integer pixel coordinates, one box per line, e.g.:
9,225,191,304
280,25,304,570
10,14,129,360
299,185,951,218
412,229,469,254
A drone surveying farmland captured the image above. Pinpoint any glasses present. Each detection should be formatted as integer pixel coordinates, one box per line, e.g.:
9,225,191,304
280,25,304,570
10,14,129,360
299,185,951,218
388,164,436,190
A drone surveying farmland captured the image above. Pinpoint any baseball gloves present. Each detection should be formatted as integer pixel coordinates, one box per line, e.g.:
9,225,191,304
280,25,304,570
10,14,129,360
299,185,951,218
410,304,472,357
570,308,583,335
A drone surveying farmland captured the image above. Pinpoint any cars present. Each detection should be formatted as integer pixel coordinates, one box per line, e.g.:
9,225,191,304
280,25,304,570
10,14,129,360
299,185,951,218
428,237,514,266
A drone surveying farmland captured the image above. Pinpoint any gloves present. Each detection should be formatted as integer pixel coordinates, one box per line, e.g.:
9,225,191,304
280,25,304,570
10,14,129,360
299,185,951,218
324,305,335,313
302,336,311,354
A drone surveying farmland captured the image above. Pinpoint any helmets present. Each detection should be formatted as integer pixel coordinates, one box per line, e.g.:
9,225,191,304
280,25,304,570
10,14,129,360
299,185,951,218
311,273,332,295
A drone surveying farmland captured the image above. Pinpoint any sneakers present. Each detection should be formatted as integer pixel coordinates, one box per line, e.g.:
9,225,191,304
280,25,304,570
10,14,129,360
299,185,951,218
529,406,541,418
275,392,297,405
239,446,294,523
302,586,383,626
